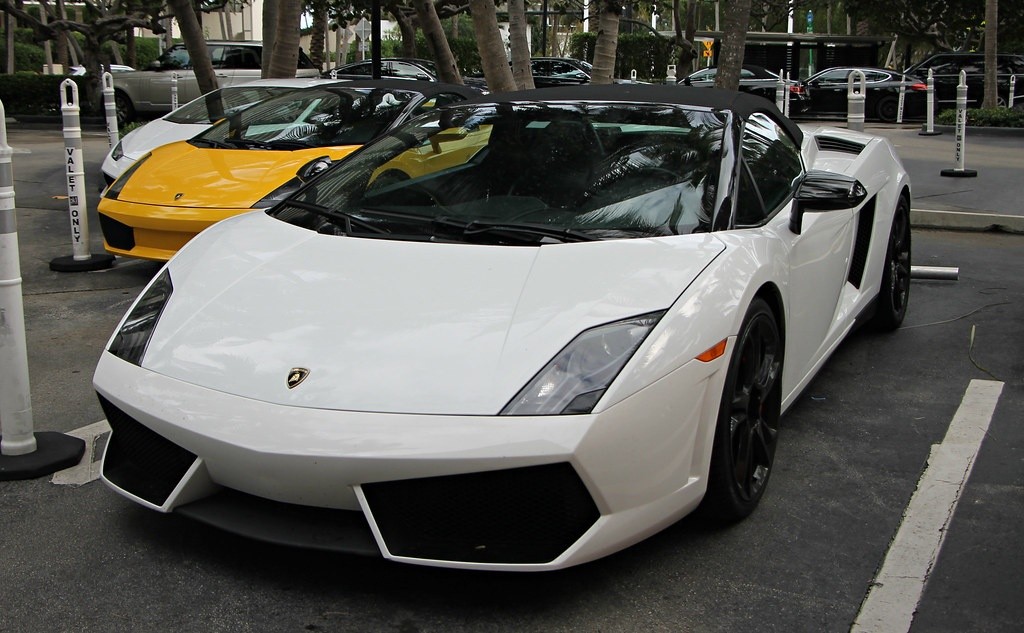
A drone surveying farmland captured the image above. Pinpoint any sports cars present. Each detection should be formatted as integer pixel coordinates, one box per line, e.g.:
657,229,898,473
90,89,911,577
99,78,353,199
99,76,495,265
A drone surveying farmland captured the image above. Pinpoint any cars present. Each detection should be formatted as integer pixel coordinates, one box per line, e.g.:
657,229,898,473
323,57,490,90
508,56,654,87
798,67,940,123
678,64,812,117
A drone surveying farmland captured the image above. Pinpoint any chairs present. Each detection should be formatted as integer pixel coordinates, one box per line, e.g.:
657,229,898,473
509,116,606,212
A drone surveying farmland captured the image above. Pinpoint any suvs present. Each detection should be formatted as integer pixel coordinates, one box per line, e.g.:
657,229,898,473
905,53,1024,107
102,39,319,118
